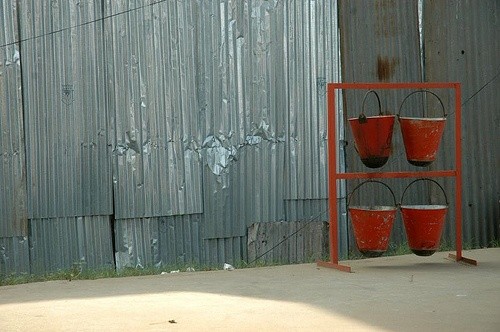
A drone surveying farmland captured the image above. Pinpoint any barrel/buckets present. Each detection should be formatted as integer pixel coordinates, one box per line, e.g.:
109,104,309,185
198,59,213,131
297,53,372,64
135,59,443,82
397,90,448,167
347,180,398,258
347,90,396,169
398,177,449,257
